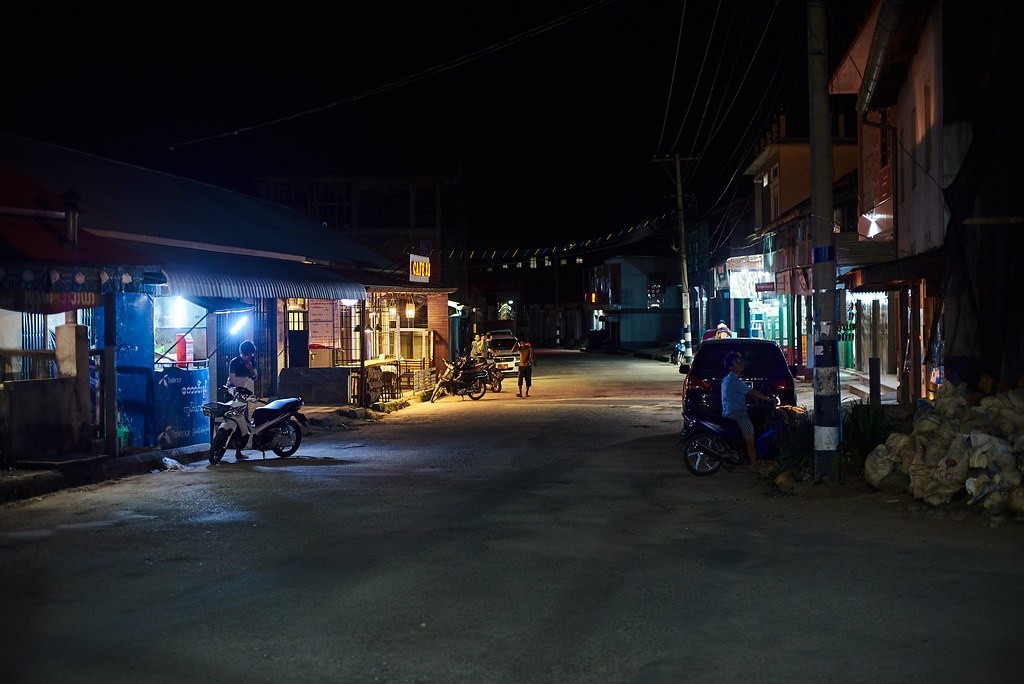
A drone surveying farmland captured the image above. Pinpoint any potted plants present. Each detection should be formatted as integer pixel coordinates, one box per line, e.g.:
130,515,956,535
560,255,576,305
760,455,797,495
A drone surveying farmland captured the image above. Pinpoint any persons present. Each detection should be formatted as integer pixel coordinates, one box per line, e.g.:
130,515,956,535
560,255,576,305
717,319,728,339
720,351,775,471
222,339,258,459
511,337,532,397
470,331,495,359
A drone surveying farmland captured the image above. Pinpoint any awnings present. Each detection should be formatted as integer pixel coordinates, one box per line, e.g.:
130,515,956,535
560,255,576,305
0,217,165,293
160,249,367,300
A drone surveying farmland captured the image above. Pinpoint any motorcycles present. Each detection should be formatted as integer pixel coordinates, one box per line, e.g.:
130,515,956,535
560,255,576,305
676,391,808,484
459,348,503,392
201,384,309,466
430,358,488,403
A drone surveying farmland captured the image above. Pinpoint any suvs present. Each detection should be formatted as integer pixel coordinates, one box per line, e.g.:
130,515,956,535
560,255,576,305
678,339,797,431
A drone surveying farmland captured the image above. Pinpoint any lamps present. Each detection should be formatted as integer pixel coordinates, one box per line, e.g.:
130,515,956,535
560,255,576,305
353,307,362,332
341,299,358,307
374,299,382,331
405,297,416,319
388,306,397,321
365,299,374,333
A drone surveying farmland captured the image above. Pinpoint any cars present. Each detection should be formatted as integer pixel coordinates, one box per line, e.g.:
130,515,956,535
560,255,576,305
483,335,523,376
